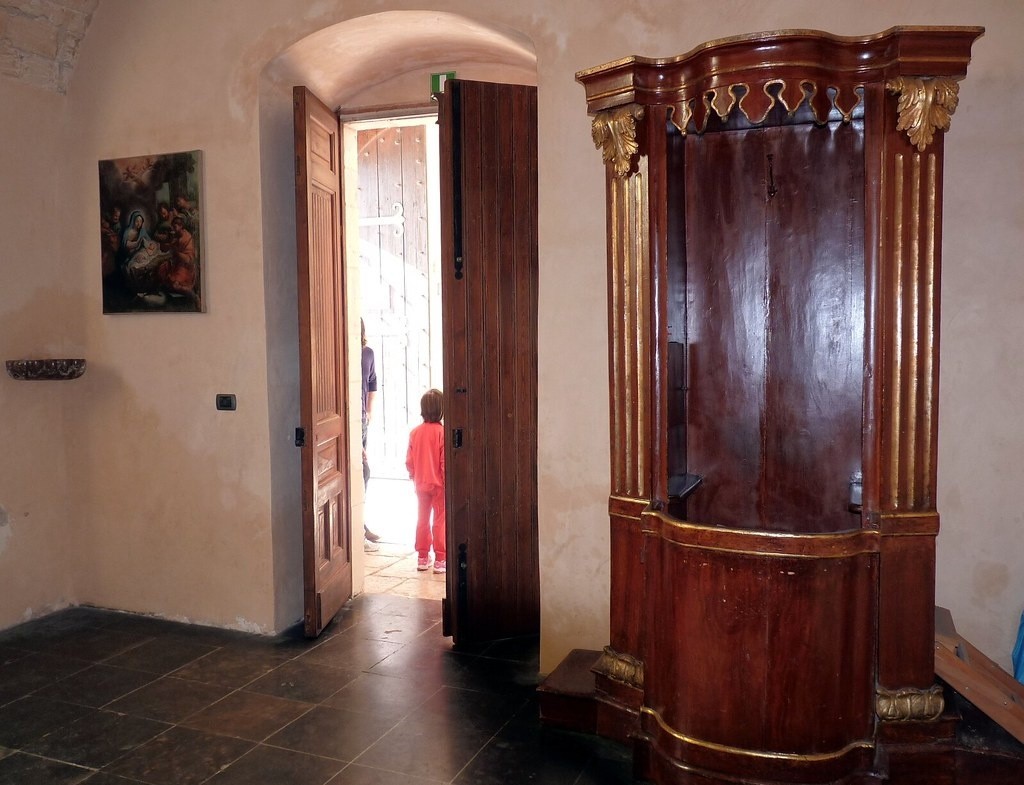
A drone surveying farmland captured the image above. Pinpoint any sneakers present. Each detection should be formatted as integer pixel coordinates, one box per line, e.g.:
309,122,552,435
417,554,432,571
364,535,380,552
364,524,381,541
433,559,447,574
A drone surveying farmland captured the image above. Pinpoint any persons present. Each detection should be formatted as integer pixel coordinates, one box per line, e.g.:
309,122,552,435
405,388,447,574
360,318,381,551
